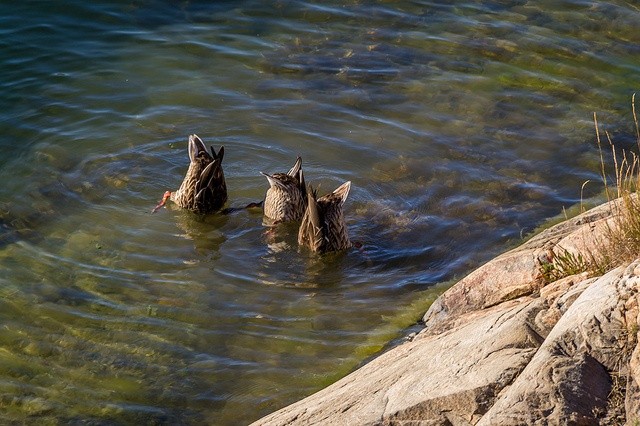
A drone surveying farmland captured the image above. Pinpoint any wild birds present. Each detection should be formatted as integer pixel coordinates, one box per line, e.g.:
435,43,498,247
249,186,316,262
259,155,309,224
152,132,228,211
298,180,353,252
